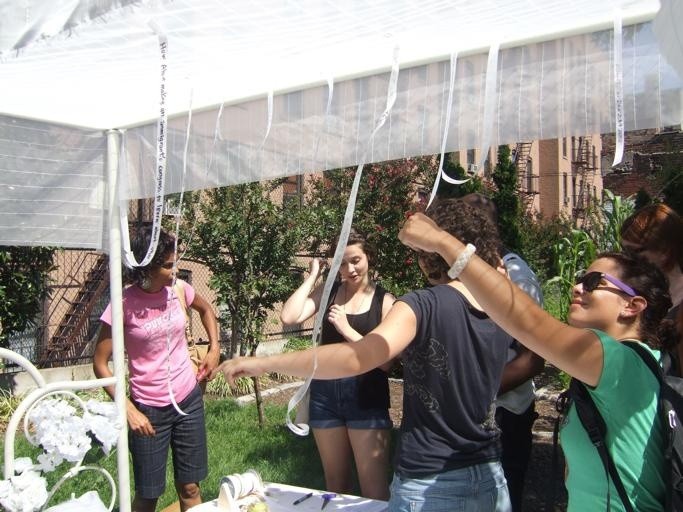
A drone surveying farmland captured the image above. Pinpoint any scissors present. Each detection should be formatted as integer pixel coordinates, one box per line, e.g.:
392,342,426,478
321,493,336,510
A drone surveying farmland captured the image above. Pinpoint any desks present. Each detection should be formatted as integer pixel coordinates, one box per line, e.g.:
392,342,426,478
186,482,390,512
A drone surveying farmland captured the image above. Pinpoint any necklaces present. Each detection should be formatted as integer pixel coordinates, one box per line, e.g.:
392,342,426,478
345,281,372,328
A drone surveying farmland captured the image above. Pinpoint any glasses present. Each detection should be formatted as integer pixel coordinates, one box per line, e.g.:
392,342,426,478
571,271,638,297
619,242,648,255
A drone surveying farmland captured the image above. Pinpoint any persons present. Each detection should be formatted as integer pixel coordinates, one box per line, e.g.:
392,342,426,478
619,203,683,379
281,225,398,503
398,211,683,512
463,192,545,512
93,220,220,512
208,199,513,512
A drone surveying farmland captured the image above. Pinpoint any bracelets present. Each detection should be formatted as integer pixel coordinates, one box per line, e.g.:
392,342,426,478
448,243,476,280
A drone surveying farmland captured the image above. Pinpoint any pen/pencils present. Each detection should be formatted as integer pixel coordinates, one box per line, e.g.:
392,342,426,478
293,492,312,505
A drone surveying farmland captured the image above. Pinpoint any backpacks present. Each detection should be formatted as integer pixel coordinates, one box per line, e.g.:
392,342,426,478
570,340,683,511
